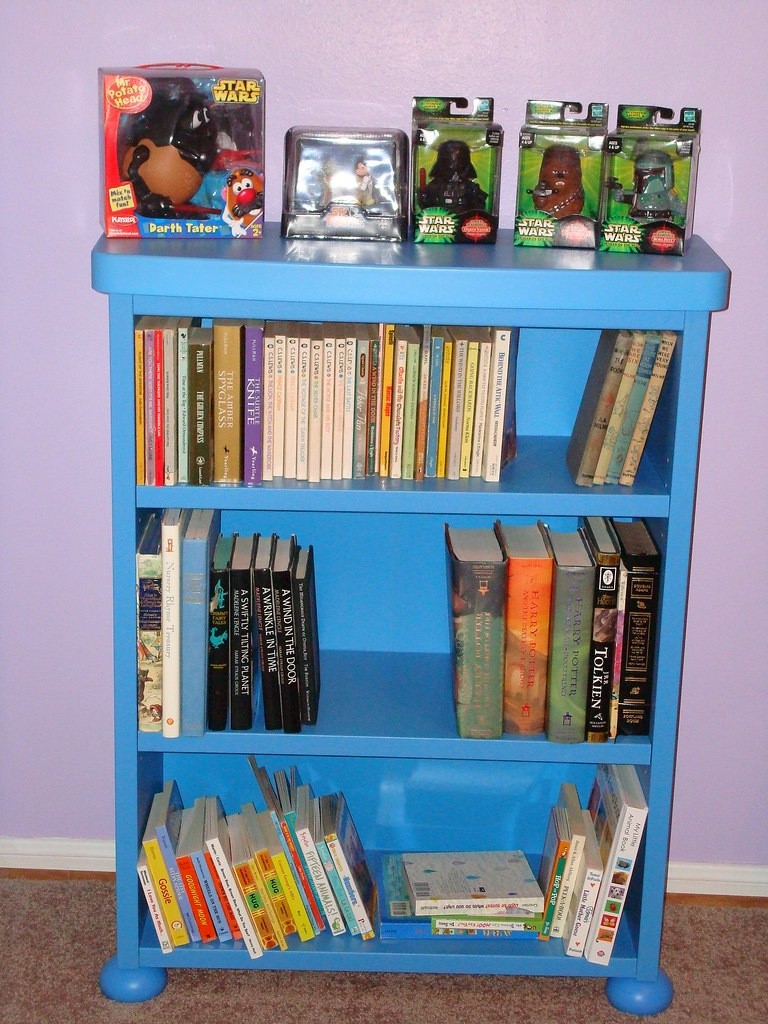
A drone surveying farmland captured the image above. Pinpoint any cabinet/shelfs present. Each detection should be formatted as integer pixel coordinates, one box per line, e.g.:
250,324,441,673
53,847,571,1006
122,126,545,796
91,235,734,1014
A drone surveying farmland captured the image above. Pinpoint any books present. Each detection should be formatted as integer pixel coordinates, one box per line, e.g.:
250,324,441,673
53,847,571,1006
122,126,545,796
564,328,677,489
443,516,661,744
134,316,520,485
135,509,320,736
137,754,649,968
